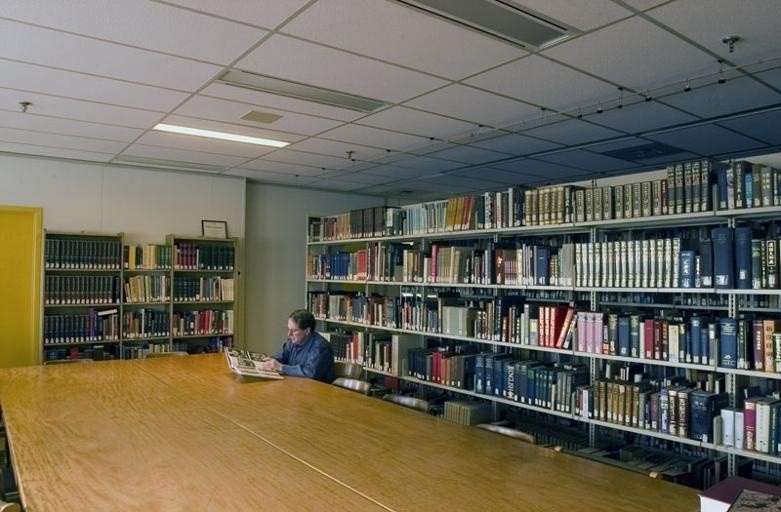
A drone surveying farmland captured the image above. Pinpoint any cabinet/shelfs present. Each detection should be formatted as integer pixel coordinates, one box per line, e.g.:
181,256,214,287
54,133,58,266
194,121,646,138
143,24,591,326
306,187,516,431
41,229,238,366
517,154,780,489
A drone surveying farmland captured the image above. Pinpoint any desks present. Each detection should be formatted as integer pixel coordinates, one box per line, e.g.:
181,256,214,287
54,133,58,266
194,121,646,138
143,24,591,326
3,356,697,510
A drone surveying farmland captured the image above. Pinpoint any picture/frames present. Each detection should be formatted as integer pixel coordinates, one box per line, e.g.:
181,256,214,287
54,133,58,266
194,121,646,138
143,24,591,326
202,220,228,239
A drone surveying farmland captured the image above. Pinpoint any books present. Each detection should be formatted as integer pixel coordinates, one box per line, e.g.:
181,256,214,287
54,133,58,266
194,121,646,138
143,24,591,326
224,345,284,380
40,230,234,365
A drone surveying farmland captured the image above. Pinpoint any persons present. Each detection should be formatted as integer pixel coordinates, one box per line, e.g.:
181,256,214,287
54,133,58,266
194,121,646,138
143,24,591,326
262,308,335,384
